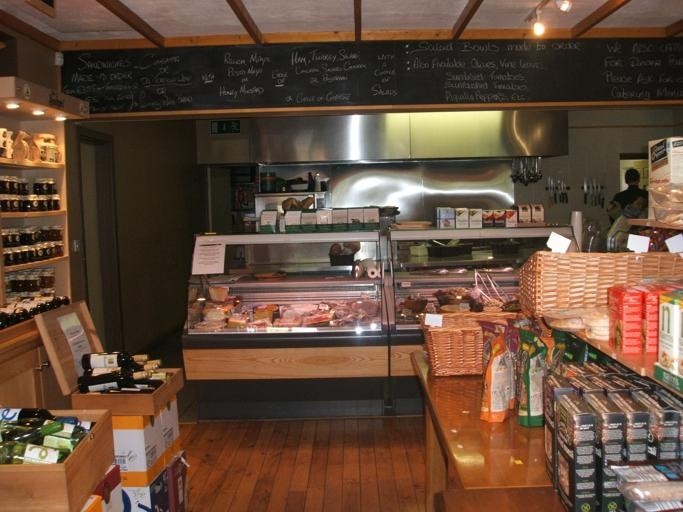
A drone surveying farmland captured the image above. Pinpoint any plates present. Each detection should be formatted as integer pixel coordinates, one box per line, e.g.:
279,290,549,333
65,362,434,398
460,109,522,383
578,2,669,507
390,220,432,230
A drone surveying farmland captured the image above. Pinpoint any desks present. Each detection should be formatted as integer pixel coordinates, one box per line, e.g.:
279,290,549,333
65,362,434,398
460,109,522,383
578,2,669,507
407,345,570,511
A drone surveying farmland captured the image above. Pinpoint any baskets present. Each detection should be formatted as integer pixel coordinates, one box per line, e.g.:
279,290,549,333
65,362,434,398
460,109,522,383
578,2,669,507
515,249,682,321
416,309,532,379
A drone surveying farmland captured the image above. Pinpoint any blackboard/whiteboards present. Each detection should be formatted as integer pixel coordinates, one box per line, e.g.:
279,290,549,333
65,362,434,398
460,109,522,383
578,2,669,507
55,37,683,121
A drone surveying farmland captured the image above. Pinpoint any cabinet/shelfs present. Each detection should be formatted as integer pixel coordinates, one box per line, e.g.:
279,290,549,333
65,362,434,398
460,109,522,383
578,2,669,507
182,223,389,421
0,157,71,332
545,216,683,406
389,229,579,415
0,346,70,410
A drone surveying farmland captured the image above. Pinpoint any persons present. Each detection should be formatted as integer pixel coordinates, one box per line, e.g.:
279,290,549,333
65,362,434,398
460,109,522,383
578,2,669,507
608,167,648,227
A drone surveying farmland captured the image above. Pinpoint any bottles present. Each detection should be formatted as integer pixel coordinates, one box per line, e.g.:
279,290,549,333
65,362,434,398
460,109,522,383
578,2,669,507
0,294,73,331
78,350,173,394
0,407,97,464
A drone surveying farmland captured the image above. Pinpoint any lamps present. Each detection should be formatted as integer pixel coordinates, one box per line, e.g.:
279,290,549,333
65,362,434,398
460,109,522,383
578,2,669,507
550,0,573,15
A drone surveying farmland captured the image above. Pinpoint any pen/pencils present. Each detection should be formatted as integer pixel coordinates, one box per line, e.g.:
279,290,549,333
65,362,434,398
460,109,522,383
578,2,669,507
201,232,217,235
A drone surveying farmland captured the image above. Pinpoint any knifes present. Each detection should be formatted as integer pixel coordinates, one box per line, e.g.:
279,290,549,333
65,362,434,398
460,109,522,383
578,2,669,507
583,175,605,208
547,175,569,208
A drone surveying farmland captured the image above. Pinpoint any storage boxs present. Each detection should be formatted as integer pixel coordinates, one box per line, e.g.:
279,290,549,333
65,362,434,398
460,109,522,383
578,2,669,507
80,393,188,512
420,314,484,377
